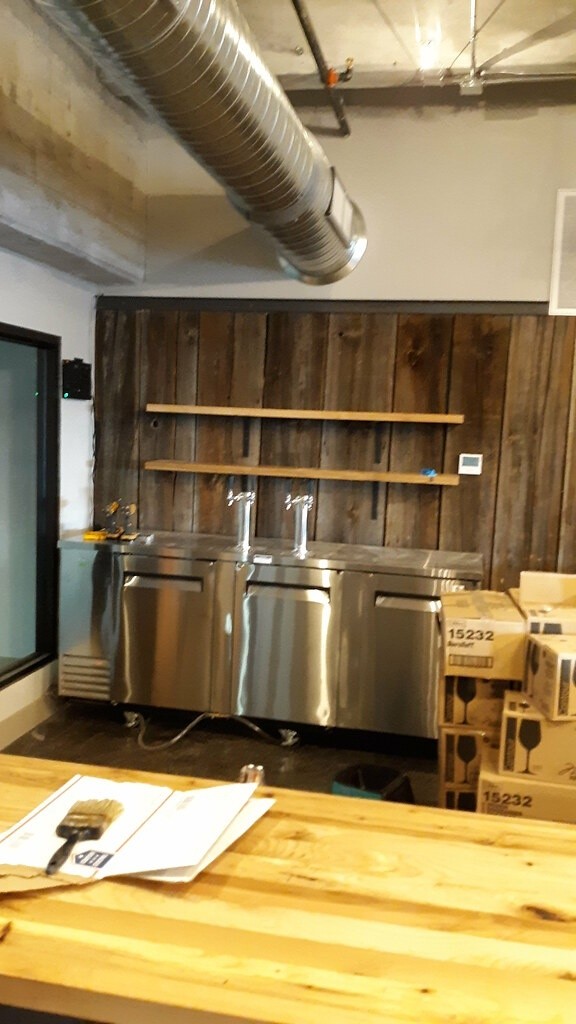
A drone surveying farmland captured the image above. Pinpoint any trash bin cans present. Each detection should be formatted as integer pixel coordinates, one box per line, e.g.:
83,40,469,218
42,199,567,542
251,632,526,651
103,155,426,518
331,764,409,801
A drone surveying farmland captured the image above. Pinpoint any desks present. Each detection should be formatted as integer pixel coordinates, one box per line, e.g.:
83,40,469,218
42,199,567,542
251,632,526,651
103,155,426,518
0,749,575,1023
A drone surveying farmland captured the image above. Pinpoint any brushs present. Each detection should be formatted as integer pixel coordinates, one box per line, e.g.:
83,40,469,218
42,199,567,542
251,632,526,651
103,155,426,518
46,799,125,875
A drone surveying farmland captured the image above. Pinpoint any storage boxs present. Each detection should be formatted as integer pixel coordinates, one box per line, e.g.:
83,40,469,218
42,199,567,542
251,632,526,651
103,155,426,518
435,571,576,826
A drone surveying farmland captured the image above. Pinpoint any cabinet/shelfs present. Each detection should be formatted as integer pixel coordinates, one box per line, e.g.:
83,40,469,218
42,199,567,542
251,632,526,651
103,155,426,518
144,404,465,485
